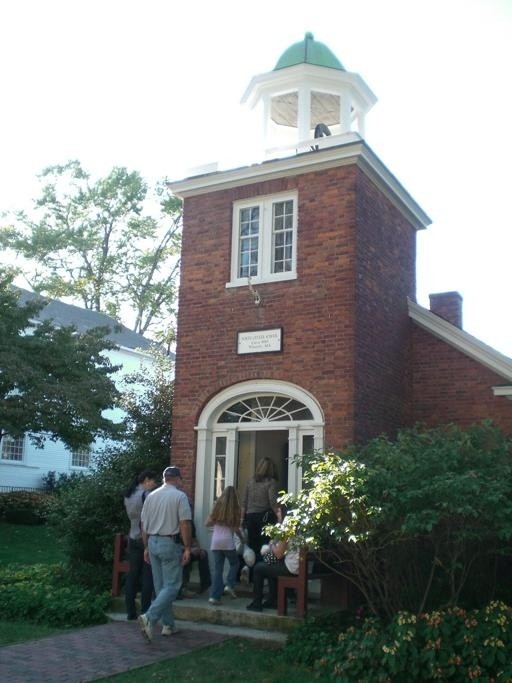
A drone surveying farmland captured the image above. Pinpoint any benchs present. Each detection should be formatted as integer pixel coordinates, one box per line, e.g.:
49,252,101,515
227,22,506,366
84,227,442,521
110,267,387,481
112,532,130,594
277,546,333,618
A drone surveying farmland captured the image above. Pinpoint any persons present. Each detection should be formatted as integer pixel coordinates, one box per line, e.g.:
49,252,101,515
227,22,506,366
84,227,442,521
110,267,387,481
239,454,284,586
173,501,211,601
204,485,246,605
232,518,248,584
246,502,301,611
123,467,158,620
137,465,193,644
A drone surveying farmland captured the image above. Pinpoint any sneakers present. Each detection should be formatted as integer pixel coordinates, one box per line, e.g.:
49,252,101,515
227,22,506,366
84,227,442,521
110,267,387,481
224,585,238,599
137,614,154,643
246,597,263,612
262,594,280,609
208,598,224,607
160,623,182,636
239,565,249,585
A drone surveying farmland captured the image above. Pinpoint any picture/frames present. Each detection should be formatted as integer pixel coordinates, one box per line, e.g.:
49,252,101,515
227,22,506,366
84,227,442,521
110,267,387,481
235,326,284,357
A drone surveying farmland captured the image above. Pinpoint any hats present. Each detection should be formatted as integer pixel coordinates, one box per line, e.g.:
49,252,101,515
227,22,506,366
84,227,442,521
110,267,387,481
163,466,182,481
